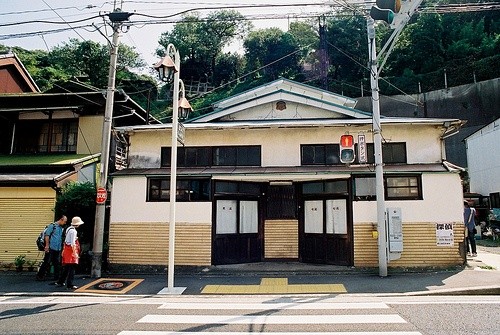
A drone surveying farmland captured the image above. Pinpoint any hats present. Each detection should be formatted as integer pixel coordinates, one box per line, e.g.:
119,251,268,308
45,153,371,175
71,216,84,226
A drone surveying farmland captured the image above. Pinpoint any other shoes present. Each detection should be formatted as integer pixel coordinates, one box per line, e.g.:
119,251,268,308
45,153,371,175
55,282,66,286
473,253,477,257
73,285,78,288
467,252,470,256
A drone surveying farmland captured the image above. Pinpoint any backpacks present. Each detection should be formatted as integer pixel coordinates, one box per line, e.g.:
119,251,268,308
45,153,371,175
36,222,56,251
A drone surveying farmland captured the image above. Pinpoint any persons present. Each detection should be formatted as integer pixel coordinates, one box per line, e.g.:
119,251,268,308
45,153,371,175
37,214,67,280
464,200,478,256
56,216,85,289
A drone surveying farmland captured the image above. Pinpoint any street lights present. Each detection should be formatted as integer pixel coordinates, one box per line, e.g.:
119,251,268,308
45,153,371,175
154,43,194,293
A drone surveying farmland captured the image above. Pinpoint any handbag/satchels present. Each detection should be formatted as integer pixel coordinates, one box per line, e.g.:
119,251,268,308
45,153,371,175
464,227,468,238
62,242,79,264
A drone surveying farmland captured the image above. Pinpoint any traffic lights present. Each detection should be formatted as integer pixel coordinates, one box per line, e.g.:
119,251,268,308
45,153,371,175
339,134,356,164
370,0,415,31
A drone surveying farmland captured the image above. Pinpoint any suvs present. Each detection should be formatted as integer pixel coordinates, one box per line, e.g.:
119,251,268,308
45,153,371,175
463,192,490,225
